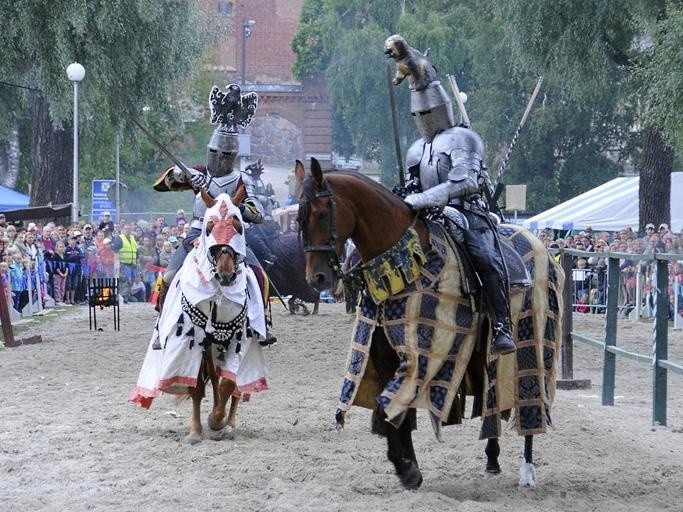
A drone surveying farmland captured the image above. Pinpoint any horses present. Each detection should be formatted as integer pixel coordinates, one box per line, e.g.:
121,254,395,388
181,184,260,446
293,155,559,491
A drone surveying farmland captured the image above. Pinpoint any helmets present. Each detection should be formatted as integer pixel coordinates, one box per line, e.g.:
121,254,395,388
207,129,238,177
410,80,455,144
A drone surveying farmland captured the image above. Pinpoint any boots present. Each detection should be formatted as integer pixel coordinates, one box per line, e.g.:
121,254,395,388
257,332,276,346
486,274,516,349
152,279,169,350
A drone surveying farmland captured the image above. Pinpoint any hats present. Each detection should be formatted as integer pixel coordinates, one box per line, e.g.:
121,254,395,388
578,231,589,238
0,214,5,218
83,223,92,230
104,211,111,216
658,224,669,232
169,237,177,242
72,231,82,239
645,223,655,228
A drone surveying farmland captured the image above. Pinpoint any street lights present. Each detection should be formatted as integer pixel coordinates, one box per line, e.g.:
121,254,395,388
66,61,85,223
241,19,255,83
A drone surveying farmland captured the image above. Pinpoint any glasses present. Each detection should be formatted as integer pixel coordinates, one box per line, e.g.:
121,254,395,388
540,235,546,238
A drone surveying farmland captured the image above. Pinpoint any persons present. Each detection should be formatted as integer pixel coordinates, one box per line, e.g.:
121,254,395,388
256,211,363,303
392,80,518,356
0,208,191,327
539,222,683,321
152,129,278,350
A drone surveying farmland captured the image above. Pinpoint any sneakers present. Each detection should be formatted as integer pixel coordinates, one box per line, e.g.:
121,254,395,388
55,300,86,306
